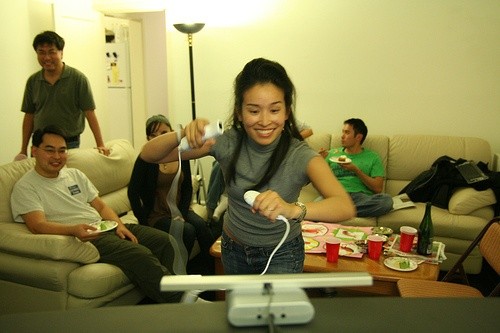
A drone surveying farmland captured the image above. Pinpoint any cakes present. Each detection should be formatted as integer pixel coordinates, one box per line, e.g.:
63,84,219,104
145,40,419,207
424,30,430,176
338,156,346,161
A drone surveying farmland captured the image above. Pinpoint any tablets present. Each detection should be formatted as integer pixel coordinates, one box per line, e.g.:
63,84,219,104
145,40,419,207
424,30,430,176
456,159,489,184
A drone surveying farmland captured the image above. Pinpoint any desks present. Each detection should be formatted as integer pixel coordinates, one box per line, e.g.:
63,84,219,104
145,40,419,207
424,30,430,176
209,232,441,300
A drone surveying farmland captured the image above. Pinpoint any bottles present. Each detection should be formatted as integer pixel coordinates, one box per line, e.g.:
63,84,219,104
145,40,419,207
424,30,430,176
419,201,434,258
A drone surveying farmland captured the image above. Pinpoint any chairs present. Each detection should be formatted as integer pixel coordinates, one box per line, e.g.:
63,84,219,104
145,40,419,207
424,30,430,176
395,216,500,298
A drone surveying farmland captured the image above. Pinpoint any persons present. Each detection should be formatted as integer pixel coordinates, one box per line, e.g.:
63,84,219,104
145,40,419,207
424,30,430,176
140,57,357,274
14,31,110,161
317,118,394,218
127,114,214,276
10,125,188,304
205,117,314,219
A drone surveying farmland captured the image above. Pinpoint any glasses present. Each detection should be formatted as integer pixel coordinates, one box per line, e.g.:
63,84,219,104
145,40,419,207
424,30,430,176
36,146,68,155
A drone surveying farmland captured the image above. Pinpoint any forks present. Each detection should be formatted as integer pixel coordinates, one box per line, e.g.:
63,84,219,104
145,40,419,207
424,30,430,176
319,149,343,155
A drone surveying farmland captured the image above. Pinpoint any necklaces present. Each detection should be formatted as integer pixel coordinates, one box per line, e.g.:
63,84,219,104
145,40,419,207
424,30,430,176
164,163,168,169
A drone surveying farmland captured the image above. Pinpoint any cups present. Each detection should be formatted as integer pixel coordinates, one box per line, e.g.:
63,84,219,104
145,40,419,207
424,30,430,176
398,225,417,253
325,240,339,265
366,235,387,263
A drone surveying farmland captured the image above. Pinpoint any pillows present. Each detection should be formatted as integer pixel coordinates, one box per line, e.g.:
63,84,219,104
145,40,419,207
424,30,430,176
448,183,496,215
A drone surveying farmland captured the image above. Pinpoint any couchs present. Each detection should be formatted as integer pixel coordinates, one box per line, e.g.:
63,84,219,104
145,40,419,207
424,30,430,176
298,132,496,275
0,137,145,306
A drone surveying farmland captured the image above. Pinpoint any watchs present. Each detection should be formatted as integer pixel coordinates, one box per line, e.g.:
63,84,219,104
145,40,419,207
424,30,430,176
290,202,306,224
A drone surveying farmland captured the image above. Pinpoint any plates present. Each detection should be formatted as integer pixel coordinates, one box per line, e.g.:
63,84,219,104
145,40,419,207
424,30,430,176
384,256,418,274
371,227,395,237
328,157,352,166
85,218,118,238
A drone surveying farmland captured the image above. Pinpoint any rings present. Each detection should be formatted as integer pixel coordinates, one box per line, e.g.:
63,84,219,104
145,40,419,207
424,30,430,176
268,208,275,211
